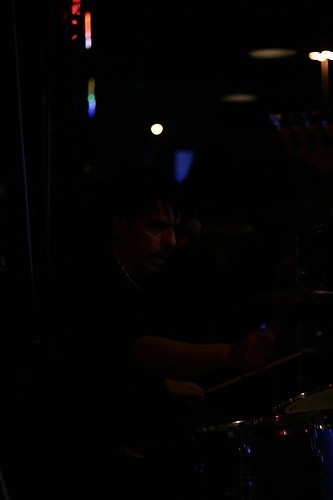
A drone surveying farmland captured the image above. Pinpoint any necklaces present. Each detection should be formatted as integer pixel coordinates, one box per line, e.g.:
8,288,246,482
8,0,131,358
110,252,143,291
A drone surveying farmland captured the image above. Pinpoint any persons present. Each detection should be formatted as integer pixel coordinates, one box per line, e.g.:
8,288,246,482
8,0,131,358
2,181,288,500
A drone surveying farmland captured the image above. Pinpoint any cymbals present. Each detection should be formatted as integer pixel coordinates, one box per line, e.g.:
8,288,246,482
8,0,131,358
285,387,333,414
192,417,262,431
250,286,333,306
